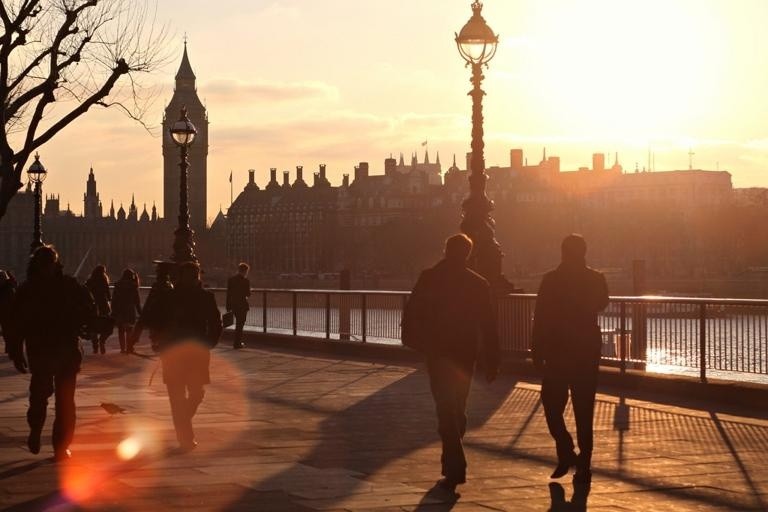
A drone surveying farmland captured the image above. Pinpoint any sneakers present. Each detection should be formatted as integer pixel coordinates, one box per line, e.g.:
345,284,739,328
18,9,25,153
551,452,577,478
54,445,72,461
182,435,198,452
578,453,592,484
440,461,467,484
28,429,40,455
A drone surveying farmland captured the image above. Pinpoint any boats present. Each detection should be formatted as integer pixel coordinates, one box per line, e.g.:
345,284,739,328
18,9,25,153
600,293,727,318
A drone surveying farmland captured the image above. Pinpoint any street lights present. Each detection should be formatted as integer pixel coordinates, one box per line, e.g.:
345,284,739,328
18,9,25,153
165,105,201,287
451,0,520,296
26,150,52,253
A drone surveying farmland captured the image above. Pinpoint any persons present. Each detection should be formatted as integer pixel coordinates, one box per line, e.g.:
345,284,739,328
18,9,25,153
401,233,500,484
5,245,100,462
226,263,251,348
158,261,222,450
0,265,173,354
533,233,609,484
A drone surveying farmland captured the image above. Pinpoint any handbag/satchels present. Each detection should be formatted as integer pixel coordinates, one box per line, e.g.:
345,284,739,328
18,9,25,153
223,312,234,328
